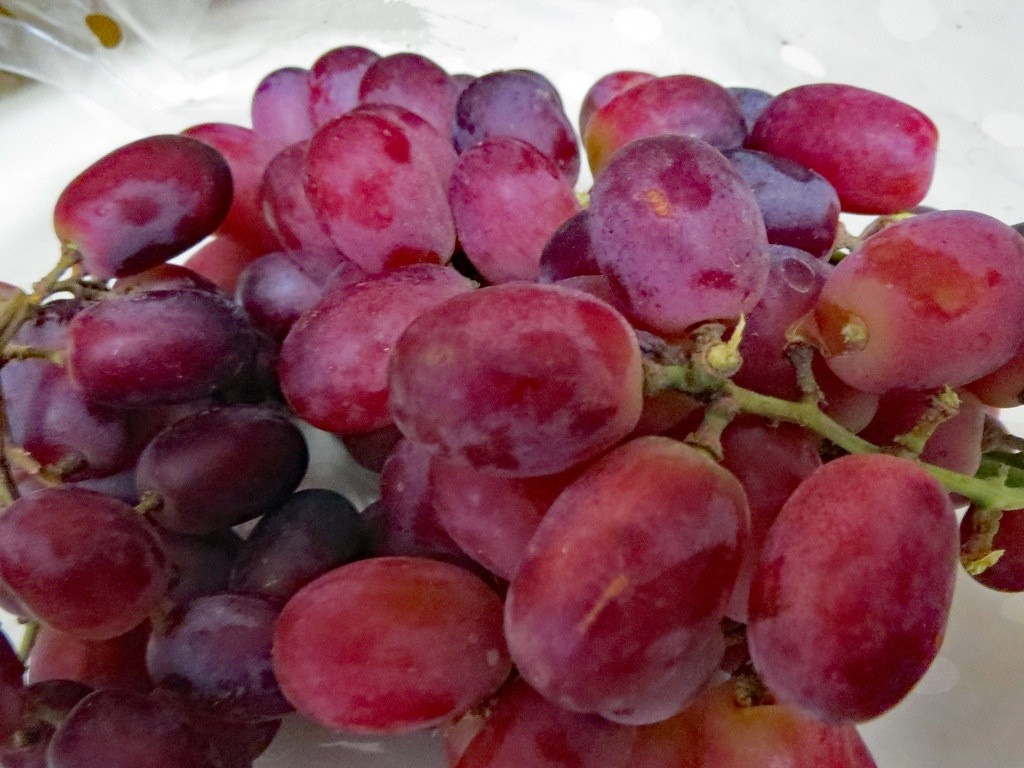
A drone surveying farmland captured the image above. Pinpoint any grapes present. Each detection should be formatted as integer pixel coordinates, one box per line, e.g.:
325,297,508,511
1,47,1024,768
